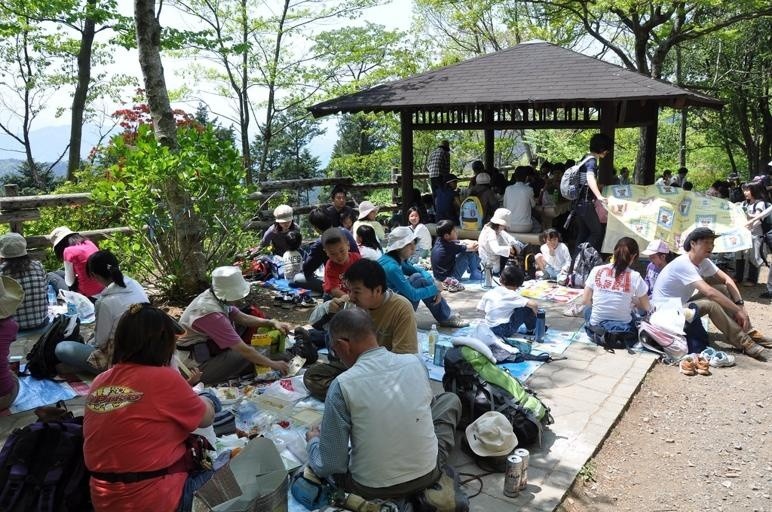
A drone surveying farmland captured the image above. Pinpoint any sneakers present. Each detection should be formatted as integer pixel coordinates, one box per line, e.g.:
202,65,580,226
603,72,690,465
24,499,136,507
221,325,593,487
679,333,771,375
733,280,772,298
294,327,318,364
441,318,470,328
442,277,465,292
274,291,317,309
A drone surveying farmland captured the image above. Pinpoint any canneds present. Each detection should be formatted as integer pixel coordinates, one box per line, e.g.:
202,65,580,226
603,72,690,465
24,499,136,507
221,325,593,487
514,449,529,489
504,455,523,497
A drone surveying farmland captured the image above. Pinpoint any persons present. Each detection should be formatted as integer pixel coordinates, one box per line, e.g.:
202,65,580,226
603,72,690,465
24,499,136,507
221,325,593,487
0,317,19,411
429,133,772,361
54,252,150,376
303,259,419,402
45,226,105,303
0,232,51,332
78,299,241,512
176,262,293,383
305,310,462,503
249,187,471,328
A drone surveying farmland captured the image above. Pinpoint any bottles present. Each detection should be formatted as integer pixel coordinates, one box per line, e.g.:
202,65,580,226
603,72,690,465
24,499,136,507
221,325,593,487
535,309,546,345
428,324,438,358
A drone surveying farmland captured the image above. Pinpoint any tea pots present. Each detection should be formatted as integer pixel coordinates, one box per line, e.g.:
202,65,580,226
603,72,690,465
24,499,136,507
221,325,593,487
480,266,493,289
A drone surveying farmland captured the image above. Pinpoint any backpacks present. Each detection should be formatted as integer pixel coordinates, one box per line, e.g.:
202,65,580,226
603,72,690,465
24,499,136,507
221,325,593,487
459,189,489,230
636,319,689,360
573,242,603,288
0,400,90,512
23,313,80,380
442,345,554,448
743,200,771,235
560,156,596,200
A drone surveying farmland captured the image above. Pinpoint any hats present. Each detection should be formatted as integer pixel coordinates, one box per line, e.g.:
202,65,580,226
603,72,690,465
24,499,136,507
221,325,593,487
274,204,293,223
476,173,492,184
358,201,379,220
212,266,250,302
444,174,461,183
0,275,25,319
490,208,511,226
0,233,27,258
466,411,519,457
385,226,420,254
50,226,79,250
438,140,450,151
642,226,722,255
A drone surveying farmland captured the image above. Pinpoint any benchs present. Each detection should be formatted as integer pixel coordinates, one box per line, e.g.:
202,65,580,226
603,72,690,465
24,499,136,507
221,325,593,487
424,225,544,252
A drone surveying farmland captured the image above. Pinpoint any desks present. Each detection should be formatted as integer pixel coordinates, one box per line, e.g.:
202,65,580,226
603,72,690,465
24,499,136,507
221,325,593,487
534,199,573,229
